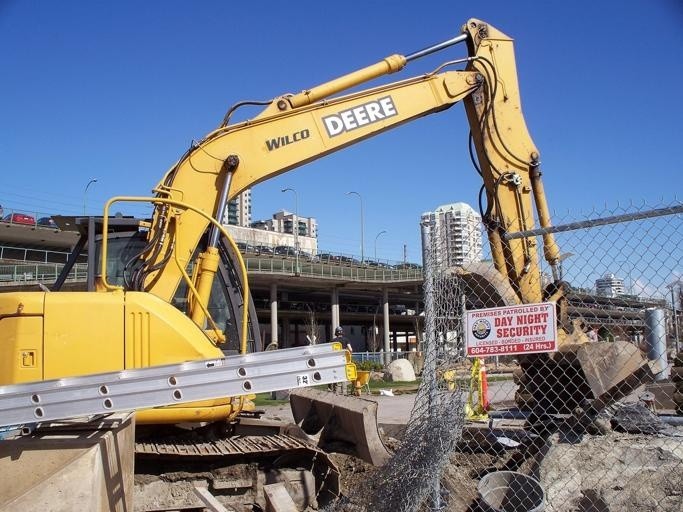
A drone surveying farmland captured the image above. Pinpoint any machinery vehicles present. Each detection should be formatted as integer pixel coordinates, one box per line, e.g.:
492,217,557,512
2,17,670,509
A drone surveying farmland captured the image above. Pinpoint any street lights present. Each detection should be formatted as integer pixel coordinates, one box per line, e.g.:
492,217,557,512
282,188,298,275
82,179,98,215
373,230,386,263
347,192,363,268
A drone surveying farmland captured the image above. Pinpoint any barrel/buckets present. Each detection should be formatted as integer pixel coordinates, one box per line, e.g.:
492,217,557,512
476,471,546,512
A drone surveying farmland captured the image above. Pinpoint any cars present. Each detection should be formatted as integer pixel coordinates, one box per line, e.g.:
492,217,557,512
3,214,57,228
237,243,422,269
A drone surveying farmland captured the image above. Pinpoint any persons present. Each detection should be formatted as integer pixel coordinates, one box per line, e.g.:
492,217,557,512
586,323,599,340
330,327,354,397
